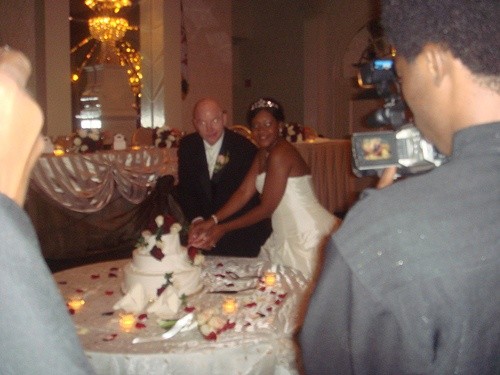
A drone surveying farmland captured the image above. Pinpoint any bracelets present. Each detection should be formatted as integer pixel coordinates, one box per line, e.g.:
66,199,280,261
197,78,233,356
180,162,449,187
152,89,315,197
211,214,217,224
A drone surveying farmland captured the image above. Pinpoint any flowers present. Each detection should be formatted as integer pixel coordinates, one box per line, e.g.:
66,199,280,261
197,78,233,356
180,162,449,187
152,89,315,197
154,124,175,148
279,123,306,144
214,154,229,172
70,127,102,153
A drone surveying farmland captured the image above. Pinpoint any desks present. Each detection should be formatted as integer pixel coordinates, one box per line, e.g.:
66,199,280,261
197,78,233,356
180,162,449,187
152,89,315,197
51,256,310,375
31,148,179,214
296,140,374,215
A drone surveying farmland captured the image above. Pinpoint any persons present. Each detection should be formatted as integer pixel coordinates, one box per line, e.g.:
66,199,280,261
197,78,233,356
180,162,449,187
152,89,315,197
0,45,95,375
299,0,500,375
188,97,343,278
176,98,268,257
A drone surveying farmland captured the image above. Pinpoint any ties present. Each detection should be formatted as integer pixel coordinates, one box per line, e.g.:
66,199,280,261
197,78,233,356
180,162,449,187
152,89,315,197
207,145,219,179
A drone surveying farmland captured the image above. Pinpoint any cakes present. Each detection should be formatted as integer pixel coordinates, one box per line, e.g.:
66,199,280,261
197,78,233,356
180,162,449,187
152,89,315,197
122,233,202,300
